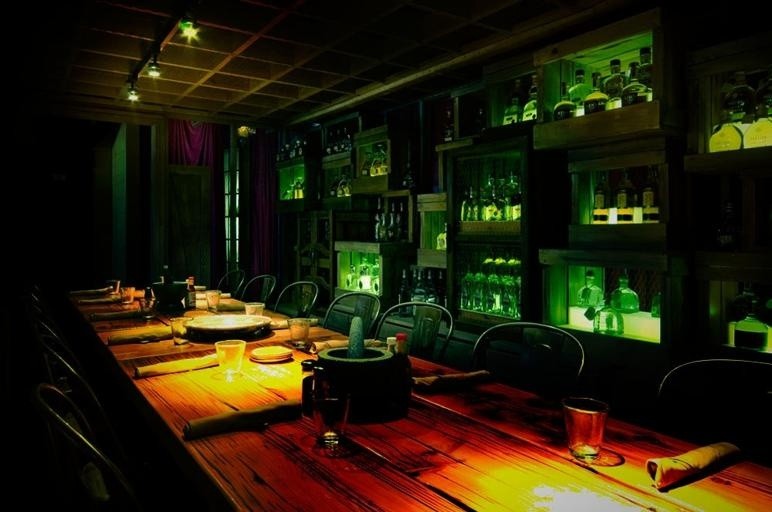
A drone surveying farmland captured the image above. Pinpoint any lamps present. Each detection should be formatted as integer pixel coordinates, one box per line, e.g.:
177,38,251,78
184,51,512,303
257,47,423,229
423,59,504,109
124,10,202,109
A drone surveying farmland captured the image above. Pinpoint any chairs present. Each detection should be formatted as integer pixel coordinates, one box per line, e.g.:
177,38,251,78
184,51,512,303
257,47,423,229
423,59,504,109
20,264,771,511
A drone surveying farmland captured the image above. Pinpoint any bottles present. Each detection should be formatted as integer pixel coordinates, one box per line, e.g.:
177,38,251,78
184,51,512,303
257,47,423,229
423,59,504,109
593,165,659,226
276,123,389,198
726,280,771,355
458,170,524,318
706,68,771,152
337,196,448,319
576,269,662,338
439,71,539,142
553,47,656,120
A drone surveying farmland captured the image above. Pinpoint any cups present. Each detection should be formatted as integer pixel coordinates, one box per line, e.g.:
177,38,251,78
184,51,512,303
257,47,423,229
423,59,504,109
558,396,610,461
306,392,350,444
104,279,313,375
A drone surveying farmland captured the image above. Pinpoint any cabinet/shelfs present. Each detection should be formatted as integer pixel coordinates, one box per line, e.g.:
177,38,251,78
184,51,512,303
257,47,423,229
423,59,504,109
274,1,771,424
